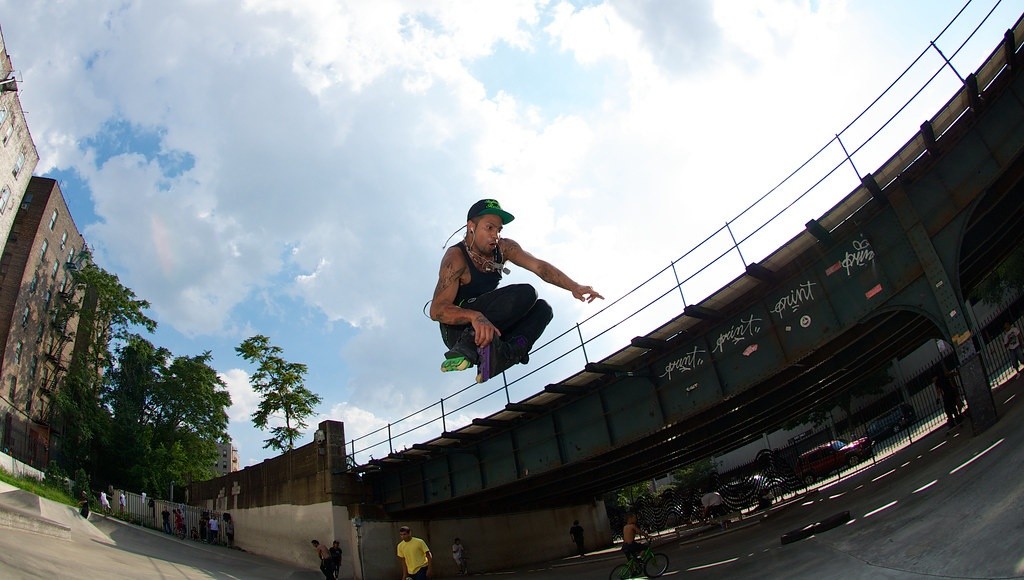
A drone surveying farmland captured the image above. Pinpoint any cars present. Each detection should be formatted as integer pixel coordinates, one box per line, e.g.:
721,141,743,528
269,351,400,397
794,436,873,486
717,475,771,507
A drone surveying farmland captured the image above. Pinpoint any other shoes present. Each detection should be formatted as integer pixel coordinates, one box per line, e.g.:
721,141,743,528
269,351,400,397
581,555,585,560
1016,372,1022,378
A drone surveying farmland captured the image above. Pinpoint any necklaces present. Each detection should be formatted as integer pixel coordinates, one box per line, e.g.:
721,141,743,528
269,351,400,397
462,237,510,275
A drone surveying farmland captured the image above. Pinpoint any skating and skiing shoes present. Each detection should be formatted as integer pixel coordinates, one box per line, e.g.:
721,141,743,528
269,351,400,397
440,328,479,373
476,332,529,383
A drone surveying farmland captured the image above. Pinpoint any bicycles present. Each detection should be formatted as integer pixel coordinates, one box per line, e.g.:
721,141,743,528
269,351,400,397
610,536,669,580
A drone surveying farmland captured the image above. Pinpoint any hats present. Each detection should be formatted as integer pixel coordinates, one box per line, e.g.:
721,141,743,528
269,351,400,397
467,199,515,225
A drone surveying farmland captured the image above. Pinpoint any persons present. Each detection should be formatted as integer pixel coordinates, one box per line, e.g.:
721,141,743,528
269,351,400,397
78,491,89,519
1002,320,1024,379
622,513,649,575
934,370,964,429
95,490,125,517
329,540,342,580
395,526,433,580
190,511,234,549
569,520,585,558
428,200,603,383
310,539,336,580
161,505,187,538
452,537,465,574
699,487,733,528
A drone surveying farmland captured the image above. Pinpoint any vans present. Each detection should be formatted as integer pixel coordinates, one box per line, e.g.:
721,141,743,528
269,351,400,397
866,403,917,441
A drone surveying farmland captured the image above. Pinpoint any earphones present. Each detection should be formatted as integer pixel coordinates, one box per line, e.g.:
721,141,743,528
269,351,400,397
471,227,474,232
